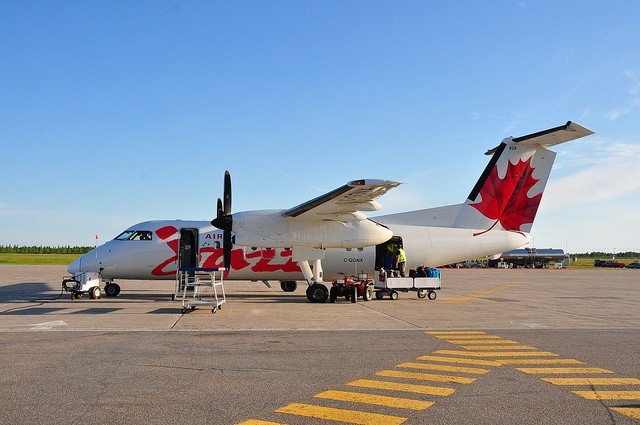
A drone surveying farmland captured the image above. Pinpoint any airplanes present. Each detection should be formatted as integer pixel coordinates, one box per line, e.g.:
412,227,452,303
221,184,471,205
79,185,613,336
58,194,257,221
66,119,596,302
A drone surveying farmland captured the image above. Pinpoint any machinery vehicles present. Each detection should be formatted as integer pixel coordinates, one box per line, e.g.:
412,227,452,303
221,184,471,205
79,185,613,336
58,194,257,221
330,269,374,304
63,271,102,301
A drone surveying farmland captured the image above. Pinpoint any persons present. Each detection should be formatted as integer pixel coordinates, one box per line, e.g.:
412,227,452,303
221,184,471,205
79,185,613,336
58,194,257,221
386,242,399,258
395,243,408,274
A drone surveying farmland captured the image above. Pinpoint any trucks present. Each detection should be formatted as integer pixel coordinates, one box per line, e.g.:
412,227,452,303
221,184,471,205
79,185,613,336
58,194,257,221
605,260,625,268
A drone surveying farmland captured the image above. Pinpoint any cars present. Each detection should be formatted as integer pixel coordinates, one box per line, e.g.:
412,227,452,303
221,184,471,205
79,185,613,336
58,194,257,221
625,263,640,269
